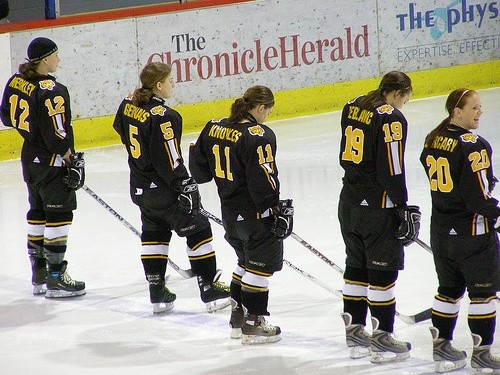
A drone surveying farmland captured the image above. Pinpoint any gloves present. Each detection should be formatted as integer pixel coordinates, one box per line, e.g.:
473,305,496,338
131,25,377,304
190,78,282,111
177,178,203,216
270,199,293,241
62,152,86,190
394,206,421,246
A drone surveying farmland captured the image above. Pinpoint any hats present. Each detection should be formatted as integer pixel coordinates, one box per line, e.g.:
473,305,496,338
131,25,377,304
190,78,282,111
27,37,58,60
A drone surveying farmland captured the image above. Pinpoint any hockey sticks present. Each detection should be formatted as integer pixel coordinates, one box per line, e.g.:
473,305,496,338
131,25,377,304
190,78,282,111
81,184,197,280
288,230,433,325
413,236,433,256
176,195,344,301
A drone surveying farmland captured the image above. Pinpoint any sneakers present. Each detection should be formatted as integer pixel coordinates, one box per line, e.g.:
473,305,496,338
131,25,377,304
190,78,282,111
45,260,86,298
369,317,411,363
29,256,47,294
197,269,231,312
429,327,467,373
146,272,176,313
471,333,500,375
241,303,282,344
229,298,244,338
340,312,372,358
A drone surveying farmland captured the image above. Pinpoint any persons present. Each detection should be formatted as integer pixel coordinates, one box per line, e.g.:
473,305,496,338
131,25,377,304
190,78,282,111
0,37,87,298
337,71,421,363
189,85,294,344
113,62,232,314
420,88,500,375
